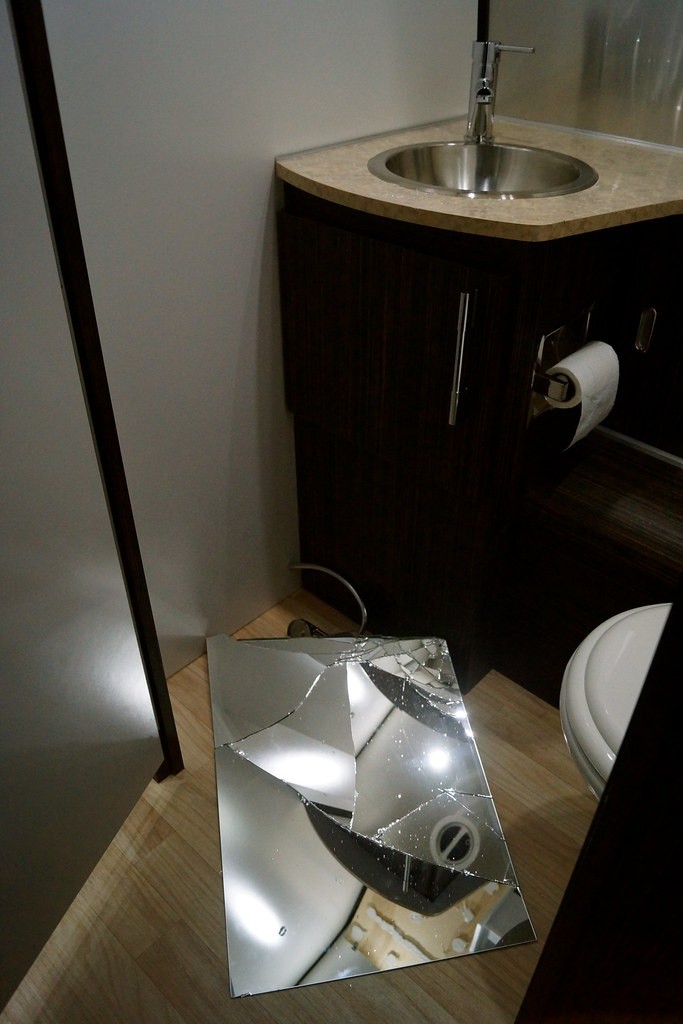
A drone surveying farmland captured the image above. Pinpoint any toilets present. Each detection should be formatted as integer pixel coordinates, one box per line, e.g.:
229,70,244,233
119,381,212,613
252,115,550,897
559,602,674,801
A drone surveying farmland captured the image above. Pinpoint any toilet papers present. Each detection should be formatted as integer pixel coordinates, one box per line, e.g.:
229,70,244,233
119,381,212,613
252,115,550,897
543,340,620,455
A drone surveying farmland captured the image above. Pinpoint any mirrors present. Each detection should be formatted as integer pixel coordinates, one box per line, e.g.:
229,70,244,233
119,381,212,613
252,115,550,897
199,636,538,995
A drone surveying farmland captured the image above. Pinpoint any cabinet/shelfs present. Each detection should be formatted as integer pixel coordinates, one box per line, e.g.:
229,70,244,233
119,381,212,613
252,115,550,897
272,215,683,664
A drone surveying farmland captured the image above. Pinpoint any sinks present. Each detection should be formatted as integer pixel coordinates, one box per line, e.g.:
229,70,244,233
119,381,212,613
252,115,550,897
368,141,601,200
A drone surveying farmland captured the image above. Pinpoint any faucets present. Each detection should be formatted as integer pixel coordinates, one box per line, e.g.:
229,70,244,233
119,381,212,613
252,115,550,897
466,39,535,142
